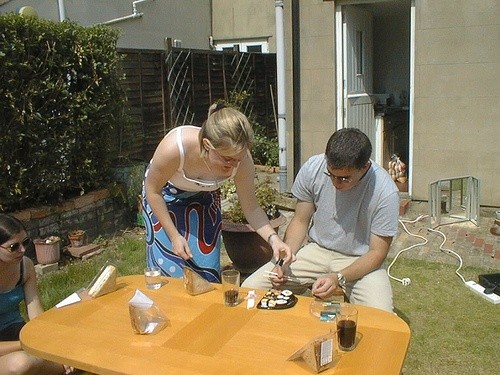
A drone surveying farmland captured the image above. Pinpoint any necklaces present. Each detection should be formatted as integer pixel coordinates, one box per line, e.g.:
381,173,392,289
201,157,220,187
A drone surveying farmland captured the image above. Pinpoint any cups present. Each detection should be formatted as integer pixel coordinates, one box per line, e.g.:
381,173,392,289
221,270,240,306
336,306,358,351
144,266,162,290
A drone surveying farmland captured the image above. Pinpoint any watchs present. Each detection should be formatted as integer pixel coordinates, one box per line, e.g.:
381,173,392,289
335,271,346,287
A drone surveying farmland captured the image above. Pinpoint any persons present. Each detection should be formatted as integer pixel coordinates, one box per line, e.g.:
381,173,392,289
142,100,297,285
0,214,76,375
240,128,400,314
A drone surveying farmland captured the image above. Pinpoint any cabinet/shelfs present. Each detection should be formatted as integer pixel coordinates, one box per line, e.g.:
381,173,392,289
376,110,408,170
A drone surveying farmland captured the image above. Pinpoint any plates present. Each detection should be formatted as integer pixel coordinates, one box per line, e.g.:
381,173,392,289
256,295,298,309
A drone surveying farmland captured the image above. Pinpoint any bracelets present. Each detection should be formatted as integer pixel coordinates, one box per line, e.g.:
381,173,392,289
268,233,278,244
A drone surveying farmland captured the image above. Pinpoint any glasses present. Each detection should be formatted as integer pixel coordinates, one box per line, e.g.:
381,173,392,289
0,236,31,252
323,162,359,183
214,149,245,164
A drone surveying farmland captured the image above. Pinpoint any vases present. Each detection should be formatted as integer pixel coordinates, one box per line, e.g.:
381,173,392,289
69,229,84,247
34,238,60,263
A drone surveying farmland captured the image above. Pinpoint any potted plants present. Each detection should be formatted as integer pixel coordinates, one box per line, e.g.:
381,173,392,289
221,175,287,275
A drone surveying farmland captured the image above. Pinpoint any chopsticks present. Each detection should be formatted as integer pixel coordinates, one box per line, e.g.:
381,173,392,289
263,270,300,283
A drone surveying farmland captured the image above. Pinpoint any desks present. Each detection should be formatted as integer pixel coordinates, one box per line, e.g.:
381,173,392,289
19,274,411,375
